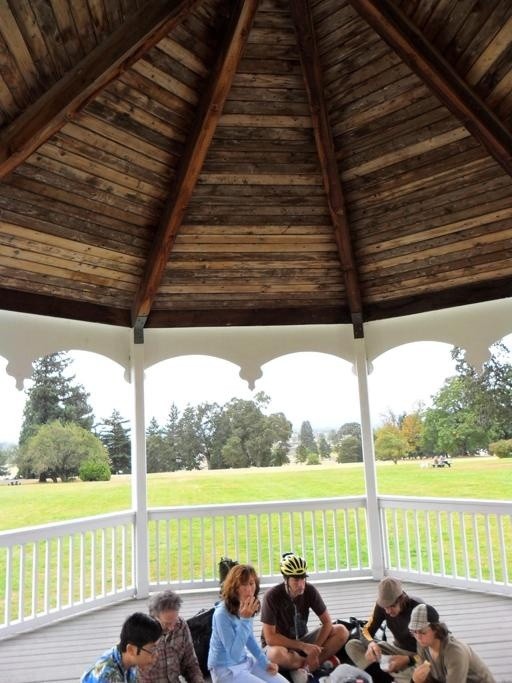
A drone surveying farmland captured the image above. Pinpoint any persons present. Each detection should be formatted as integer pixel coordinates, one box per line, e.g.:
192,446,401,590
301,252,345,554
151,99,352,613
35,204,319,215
207,562,293,683
80,611,164,683
406,602,499,683
135,588,207,682
258,550,350,683
341,576,421,683
433,455,451,467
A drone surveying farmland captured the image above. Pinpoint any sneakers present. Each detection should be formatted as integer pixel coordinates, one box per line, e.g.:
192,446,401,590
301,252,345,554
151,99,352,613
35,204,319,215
318,655,340,673
290,667,310,683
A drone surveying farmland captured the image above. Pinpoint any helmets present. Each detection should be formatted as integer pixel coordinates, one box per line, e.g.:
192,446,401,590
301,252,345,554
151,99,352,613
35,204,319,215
279,552,309,580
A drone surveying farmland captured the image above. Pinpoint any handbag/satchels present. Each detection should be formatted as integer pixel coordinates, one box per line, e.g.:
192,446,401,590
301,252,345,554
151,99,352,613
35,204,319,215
336,619,362,639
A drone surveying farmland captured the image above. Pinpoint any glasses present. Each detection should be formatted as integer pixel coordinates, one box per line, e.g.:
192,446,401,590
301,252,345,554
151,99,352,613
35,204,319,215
141,647,160,657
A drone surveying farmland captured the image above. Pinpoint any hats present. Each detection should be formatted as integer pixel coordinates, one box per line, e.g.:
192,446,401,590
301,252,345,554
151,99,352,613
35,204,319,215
408,602,440,630
375,576,403,608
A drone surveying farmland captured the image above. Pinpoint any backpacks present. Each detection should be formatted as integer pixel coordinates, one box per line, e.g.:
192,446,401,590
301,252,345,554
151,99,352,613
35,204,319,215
218,556,239,583
